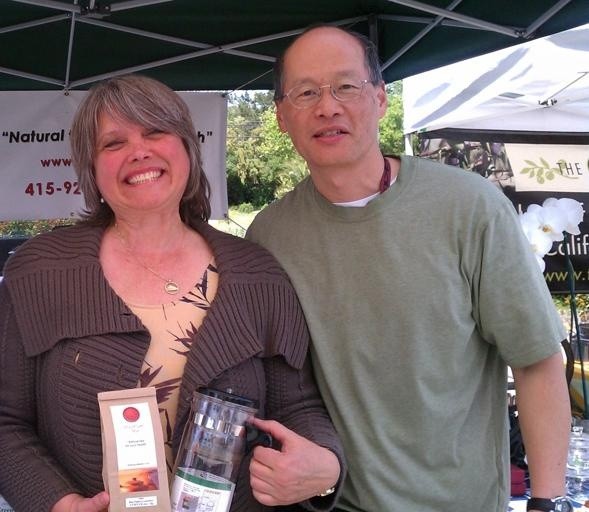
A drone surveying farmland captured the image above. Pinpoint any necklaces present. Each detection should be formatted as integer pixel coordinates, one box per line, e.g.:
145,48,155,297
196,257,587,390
115,223,189,296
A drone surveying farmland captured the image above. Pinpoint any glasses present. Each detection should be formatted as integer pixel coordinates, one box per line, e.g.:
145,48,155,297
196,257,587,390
274,76,372,111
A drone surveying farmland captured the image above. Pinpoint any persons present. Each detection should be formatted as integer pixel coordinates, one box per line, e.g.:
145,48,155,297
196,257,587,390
242,26,573,511
0,73,349,511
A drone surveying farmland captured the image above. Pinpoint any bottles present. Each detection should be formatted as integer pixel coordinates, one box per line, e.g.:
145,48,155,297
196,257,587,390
167,387,271,511
565,422,588,506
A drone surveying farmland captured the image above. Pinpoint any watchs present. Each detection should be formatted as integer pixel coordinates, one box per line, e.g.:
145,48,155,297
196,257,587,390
526,495,573,512
320,487,336,497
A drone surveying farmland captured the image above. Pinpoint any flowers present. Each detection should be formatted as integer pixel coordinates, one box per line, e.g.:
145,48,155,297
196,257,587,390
514,193,587,272
